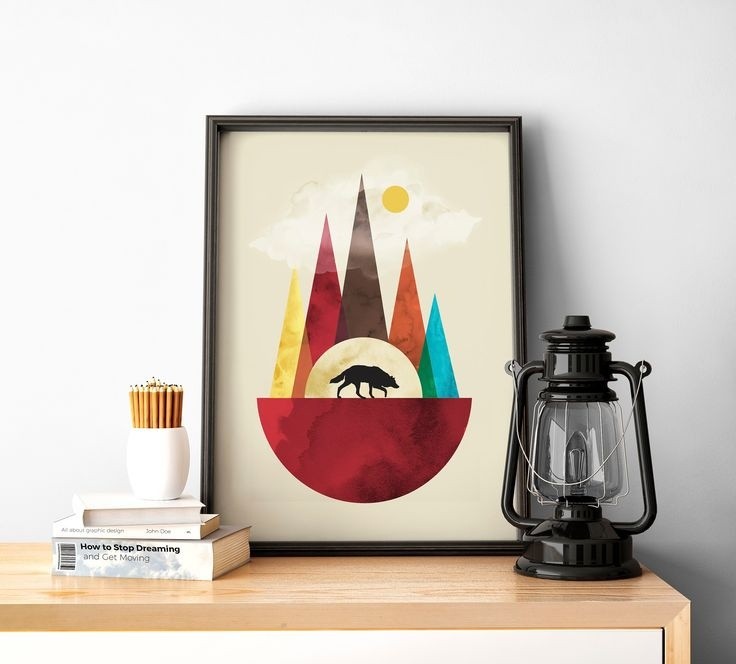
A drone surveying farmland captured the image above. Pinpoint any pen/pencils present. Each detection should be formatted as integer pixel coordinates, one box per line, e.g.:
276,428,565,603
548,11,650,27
129,376,183,428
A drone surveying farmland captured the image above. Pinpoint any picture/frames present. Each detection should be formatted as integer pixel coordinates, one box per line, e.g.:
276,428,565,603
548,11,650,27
197,112,531,560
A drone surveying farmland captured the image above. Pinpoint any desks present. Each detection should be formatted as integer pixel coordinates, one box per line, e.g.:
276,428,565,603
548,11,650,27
0,538,695,664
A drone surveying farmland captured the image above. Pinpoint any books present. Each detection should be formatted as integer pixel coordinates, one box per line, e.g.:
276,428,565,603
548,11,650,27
50,498,252,582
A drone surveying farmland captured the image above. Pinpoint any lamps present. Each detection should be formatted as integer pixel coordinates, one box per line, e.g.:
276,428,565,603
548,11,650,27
500,314,659,584
